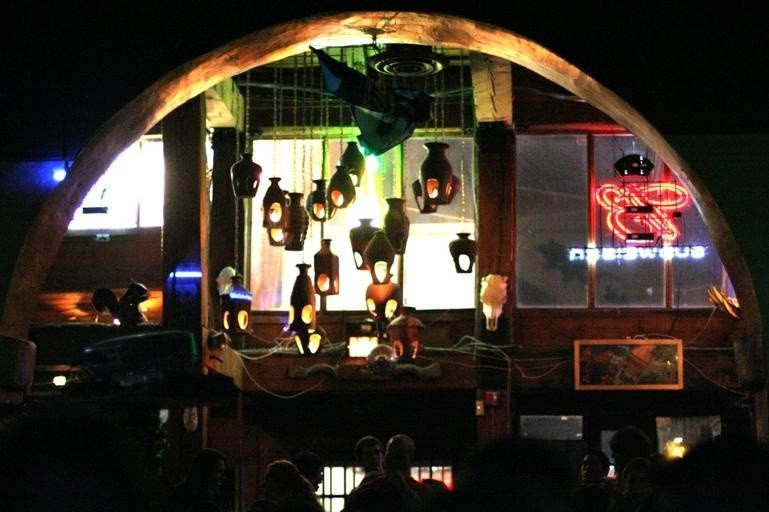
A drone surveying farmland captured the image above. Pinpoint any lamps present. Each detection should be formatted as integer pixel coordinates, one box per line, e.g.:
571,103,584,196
224,44,543,352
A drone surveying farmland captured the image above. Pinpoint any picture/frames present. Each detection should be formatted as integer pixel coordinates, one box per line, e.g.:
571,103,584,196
573,337,687,393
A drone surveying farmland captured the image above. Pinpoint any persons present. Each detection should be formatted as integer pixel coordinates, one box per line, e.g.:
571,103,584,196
111,425,322,509
341,434,446,510
450,426,676,509
